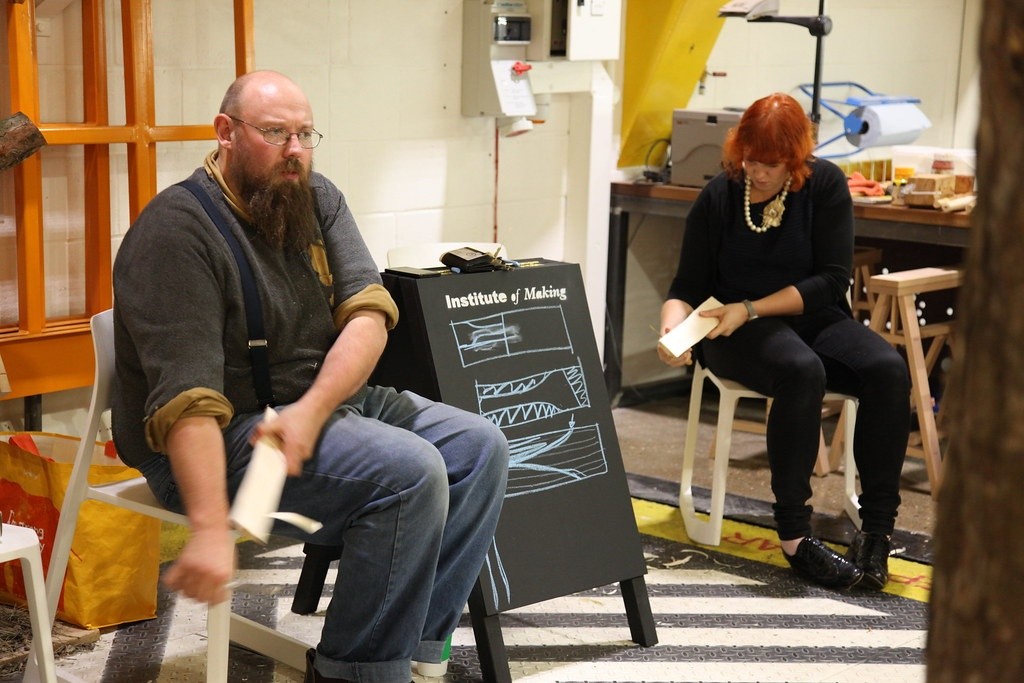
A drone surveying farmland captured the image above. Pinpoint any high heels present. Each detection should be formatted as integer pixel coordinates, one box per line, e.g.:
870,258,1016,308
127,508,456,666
781,534,865,590
845,530,891,589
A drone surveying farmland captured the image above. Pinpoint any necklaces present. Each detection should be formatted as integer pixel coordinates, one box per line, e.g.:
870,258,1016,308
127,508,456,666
744,174,793,232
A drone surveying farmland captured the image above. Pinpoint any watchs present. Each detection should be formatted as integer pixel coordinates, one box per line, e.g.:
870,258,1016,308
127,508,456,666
743,297,758,321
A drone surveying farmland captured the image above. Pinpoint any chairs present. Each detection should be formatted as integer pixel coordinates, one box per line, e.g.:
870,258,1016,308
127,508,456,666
27,308,279,682
664,285,862,546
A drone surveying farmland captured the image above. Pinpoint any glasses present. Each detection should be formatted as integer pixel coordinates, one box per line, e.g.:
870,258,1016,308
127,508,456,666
229,116,323,149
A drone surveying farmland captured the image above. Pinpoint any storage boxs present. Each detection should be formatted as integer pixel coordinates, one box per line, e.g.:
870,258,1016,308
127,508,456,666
669,107,745,191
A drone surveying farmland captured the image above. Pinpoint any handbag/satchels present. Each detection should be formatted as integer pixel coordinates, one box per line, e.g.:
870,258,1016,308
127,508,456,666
1,430,160,631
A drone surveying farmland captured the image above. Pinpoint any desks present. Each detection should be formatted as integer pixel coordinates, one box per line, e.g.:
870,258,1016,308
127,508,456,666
603,179,970,413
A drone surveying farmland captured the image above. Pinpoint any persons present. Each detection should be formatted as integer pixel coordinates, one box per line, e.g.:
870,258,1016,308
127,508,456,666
110,71,509,683
658,92,912,591
